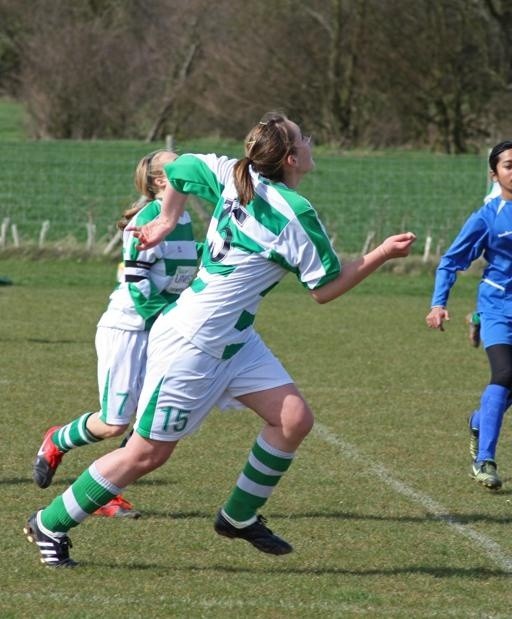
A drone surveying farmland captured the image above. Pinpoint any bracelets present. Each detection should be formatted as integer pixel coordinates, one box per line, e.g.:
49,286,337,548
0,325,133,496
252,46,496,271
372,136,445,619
380,242,391,261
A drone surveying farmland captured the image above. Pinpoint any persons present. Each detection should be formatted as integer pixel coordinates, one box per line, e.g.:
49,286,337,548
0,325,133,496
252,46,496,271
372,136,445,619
424,137,512,495
30,150,207,518
23,111,415,570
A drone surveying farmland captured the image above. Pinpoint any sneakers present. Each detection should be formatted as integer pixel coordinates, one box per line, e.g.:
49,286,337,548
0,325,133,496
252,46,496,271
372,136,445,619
90,493,141,518
33,425,67,488
468,414,479,461
214,505,292,556
22,508,77,569
468,457,502,490
